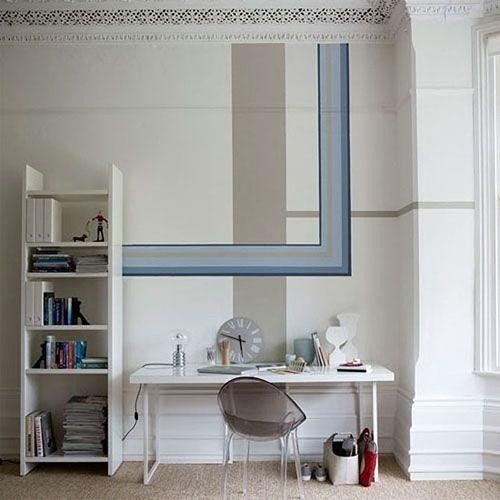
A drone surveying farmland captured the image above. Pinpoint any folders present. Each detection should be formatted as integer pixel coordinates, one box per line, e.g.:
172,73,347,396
27,198,62,243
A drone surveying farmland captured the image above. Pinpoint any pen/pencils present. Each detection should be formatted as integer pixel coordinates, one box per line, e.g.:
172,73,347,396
207,340,229,360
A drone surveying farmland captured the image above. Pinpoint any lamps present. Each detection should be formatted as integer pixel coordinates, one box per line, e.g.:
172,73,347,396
167,329,189,370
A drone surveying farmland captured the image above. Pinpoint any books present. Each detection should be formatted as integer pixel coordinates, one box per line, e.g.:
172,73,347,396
336,365,372,372
255,364,287,371
26,198,63,243
26,395,107,457
30,247,108,272
45,335,108,369
25,281,78,325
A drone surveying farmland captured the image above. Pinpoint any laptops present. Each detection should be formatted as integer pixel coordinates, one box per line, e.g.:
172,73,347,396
197,365,258,375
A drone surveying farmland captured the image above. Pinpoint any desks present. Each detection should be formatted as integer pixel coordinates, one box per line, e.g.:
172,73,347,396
129,365,394,485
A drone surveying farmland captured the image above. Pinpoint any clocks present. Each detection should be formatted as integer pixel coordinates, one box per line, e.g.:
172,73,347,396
216,317,264,365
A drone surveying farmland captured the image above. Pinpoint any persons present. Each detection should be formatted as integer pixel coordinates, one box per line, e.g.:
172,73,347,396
92,211,108,241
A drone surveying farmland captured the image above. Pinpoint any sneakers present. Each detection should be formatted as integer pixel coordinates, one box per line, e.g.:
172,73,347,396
301,463,312,481
313,462,326,482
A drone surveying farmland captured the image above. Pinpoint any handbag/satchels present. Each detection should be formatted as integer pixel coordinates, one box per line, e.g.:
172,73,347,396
356,428,378,487
323,433,360,486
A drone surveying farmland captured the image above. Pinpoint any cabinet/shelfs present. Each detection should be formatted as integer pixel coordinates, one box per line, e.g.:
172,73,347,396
20,162,125,477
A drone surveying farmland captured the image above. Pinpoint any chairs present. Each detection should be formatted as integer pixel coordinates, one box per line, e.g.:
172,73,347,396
216,376,308,499
